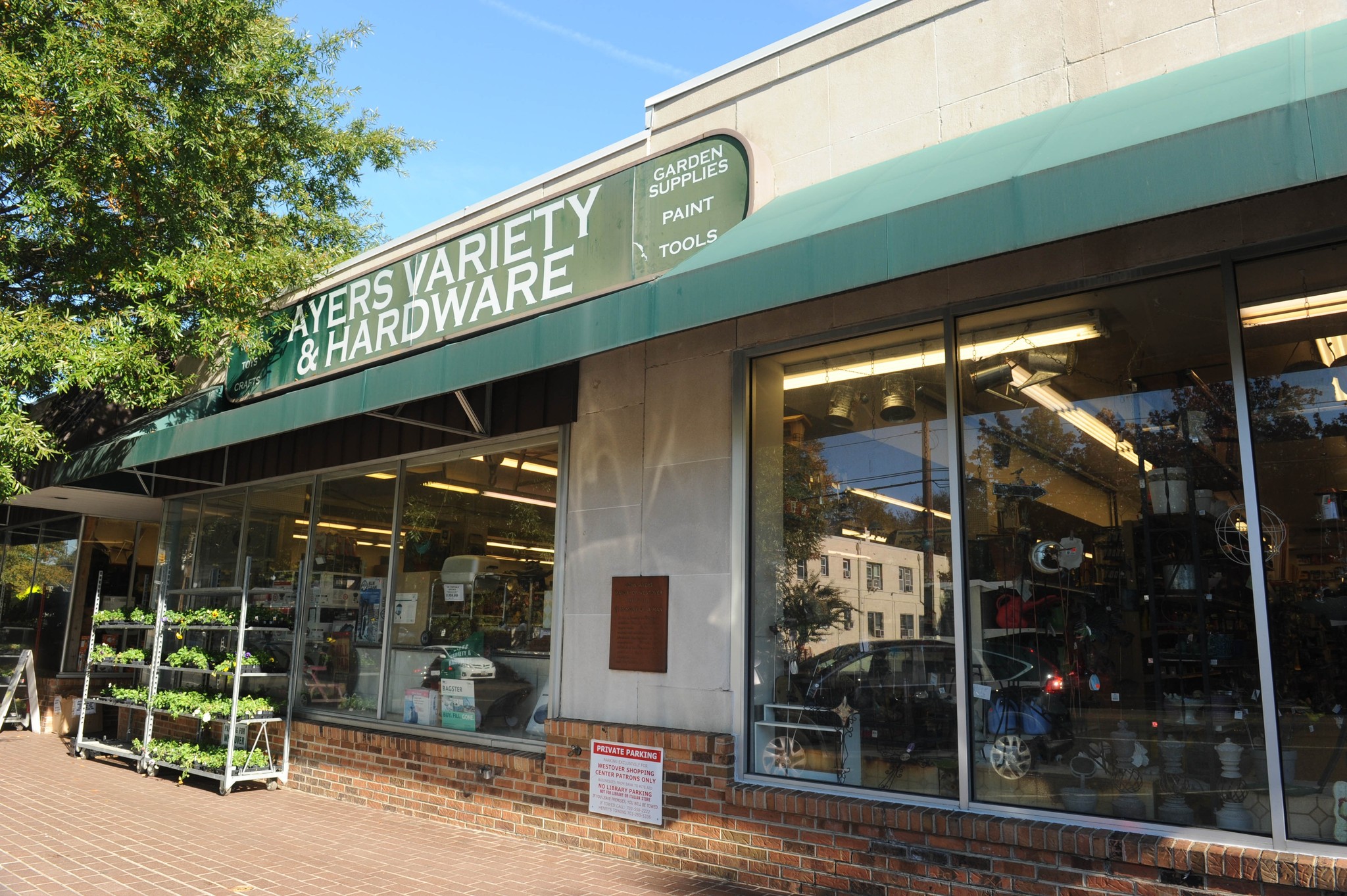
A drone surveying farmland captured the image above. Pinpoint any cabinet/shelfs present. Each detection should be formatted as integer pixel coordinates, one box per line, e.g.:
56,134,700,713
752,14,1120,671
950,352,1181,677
0,649,41,734
68,563,216,775
970,505,1287,829
143,557,304,795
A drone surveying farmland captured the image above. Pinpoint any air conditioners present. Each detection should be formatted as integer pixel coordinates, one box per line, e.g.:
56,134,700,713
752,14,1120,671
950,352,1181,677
900,629,913,638
867,579,879,589
843,570,850,579
876,629,884,637
844,620,854,630
906,585,913,592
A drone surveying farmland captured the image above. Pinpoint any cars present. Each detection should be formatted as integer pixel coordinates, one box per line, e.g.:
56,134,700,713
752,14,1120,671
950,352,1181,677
754,639,1076,791
416,645,497,684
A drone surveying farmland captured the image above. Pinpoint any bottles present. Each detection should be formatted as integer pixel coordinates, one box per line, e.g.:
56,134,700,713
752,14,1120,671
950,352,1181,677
1024,697,1052,735
988,697,1018,735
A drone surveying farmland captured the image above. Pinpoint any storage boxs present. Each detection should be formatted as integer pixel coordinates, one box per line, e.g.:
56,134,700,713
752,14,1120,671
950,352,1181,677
251,530,486,683
403,687,439,726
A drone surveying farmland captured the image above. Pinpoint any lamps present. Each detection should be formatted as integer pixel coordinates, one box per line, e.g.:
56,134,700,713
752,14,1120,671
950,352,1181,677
783,310,1112,392
1238,285,1347,329
1116,448,1154,472
970,354,1013,394
1025,344,1079,377
1054,405,1135,452
878,372,915,424
824,379,862,430
1001,355,1077,415
292,454,557,565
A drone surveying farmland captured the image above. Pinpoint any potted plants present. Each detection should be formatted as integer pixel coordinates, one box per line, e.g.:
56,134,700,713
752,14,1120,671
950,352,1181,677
90,604,291,787
51,689,104,736
0,643,28,718
